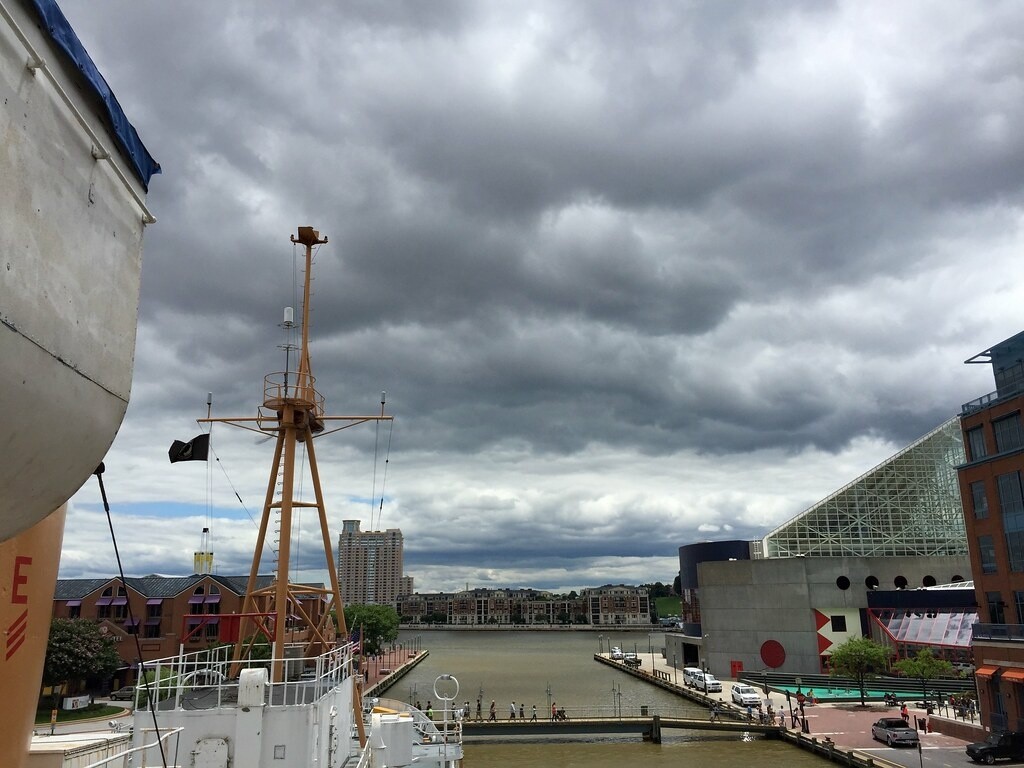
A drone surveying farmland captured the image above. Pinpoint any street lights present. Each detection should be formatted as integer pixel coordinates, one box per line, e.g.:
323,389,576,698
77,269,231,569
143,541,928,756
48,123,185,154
362,634,421,684
616,683,623,718
700,657,708,696
647,634,651,653
408,686,414,706
672,650,678,684
761,668,770,714
634,642,637,653
650,645,655,675
795,677,806,732
611,679,618,716
412,683,418,706
598,635,601,655
547,683,553,722
600,634,604,653
607,637,611,659
545,680,551,717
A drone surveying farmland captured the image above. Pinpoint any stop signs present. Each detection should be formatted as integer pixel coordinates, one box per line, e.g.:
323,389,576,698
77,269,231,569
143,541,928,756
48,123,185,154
796,693,806,703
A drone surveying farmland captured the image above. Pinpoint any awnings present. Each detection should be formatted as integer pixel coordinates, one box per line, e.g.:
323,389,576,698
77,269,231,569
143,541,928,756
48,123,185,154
318,598,330,616
188,596,221,603
267,595,303,621
94,598,127,605
188,617,219,624
66,601,81,606
123,618,161,626
975,664,1000,679
1002,668,1024,683
147,599,163,604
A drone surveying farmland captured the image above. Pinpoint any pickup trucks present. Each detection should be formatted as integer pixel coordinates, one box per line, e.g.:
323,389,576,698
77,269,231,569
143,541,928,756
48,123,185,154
872,717,921,749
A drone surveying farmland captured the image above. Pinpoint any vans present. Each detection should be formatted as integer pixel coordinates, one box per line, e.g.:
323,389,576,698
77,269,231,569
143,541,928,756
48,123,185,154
682,666,703,688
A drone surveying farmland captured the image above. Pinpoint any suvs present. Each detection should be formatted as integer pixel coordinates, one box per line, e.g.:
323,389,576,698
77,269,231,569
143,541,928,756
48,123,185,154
109,685,136,701
731,683,762,708
611,646,625,660
965,729,1024,766
691,673,722,693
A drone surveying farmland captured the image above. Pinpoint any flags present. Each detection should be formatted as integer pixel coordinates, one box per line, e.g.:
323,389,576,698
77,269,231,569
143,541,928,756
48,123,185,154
168,434,210,463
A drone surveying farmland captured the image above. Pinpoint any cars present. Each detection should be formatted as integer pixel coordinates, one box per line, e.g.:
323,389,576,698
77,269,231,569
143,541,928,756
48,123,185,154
623,652,637,665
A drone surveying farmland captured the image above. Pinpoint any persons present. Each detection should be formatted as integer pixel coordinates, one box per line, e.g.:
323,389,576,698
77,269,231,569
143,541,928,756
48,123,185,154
451,699,566,722
926,704,933,715
884,692,897,706
900,704,909,722
372,652,382,663
708,701,802,728
324,666,369,683
948,694,976,716
410,700,433,721
784,688,816,706
